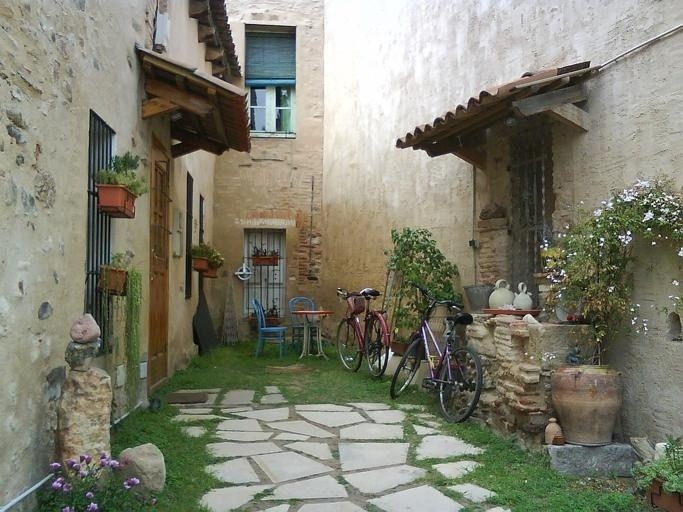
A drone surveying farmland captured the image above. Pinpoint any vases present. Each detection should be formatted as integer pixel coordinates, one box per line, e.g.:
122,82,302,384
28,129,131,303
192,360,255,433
550,367,621,446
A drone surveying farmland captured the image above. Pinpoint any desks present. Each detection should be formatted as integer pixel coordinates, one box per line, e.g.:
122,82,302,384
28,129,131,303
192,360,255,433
292,310,335,361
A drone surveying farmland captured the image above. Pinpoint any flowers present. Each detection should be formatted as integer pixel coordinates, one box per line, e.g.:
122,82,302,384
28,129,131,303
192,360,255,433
540,169,683,367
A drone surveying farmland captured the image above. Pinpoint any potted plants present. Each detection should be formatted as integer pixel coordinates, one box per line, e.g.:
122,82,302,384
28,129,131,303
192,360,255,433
201,247,224,278
93,149,150,219
189,241,212,272
382,225,464,359
630,433,683,512
248,304,281,327
248,246,281,266
97,249,142,427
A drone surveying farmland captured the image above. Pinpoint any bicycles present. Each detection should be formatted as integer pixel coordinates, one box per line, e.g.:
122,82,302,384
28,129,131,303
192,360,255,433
390,279,484,426
334,287,390,377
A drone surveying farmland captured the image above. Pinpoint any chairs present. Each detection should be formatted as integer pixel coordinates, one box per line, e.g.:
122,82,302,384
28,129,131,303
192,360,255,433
251,296,287,361
288,296,318,355
